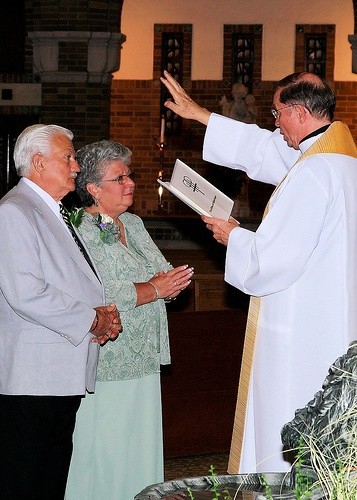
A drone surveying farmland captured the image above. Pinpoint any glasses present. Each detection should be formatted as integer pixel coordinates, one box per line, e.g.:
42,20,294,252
271,103,311,119
98,173,133,184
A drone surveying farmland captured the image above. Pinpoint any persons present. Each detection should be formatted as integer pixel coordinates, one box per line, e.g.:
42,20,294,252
64,138,195,500
161,71,357,474
0,125,124,500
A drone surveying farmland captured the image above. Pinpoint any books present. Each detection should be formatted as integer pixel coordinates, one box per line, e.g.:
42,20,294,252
155,159,241,226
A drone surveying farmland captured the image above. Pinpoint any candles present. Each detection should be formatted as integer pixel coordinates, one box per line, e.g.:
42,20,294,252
161,114,165,142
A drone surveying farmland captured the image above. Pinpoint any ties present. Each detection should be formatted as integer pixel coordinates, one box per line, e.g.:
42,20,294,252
59,203,102,284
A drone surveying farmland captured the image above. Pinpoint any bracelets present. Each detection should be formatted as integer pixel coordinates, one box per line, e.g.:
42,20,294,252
90,315,98,333
151,281,159,302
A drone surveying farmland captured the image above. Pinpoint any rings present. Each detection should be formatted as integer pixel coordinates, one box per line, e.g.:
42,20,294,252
174,282,178,286
